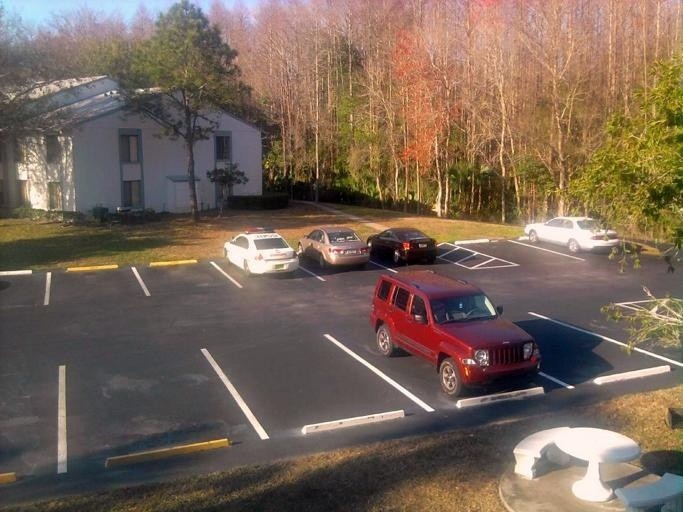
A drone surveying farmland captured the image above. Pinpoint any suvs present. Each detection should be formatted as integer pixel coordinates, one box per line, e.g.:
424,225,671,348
369,269,544,400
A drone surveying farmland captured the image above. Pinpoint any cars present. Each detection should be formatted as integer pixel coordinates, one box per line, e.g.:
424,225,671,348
222,226,300,278
366,226,438,266
297,226,371,270
522,215,620,255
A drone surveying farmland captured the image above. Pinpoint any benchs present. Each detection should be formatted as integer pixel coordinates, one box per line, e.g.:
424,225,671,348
512,426,683,511
